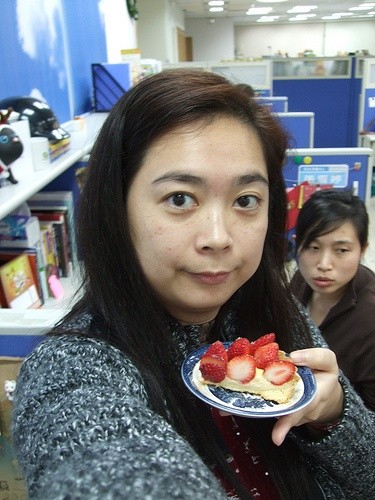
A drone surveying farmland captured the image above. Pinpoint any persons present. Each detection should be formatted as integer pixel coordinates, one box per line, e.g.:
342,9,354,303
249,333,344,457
8,69,375,500
291,187,375,412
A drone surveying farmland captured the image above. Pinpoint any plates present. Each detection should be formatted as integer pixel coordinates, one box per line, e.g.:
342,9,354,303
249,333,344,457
181,341,318,417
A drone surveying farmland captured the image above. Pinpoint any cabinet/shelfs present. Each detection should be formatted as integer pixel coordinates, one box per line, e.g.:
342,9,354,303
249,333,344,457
0,110,112,357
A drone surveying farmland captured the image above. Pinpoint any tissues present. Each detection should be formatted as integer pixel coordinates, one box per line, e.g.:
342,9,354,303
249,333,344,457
0,202,41,248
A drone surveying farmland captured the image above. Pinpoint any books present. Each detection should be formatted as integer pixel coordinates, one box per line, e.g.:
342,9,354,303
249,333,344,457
0,185,79,312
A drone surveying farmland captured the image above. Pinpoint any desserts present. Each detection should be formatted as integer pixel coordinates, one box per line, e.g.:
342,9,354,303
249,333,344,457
198,334,296,404
